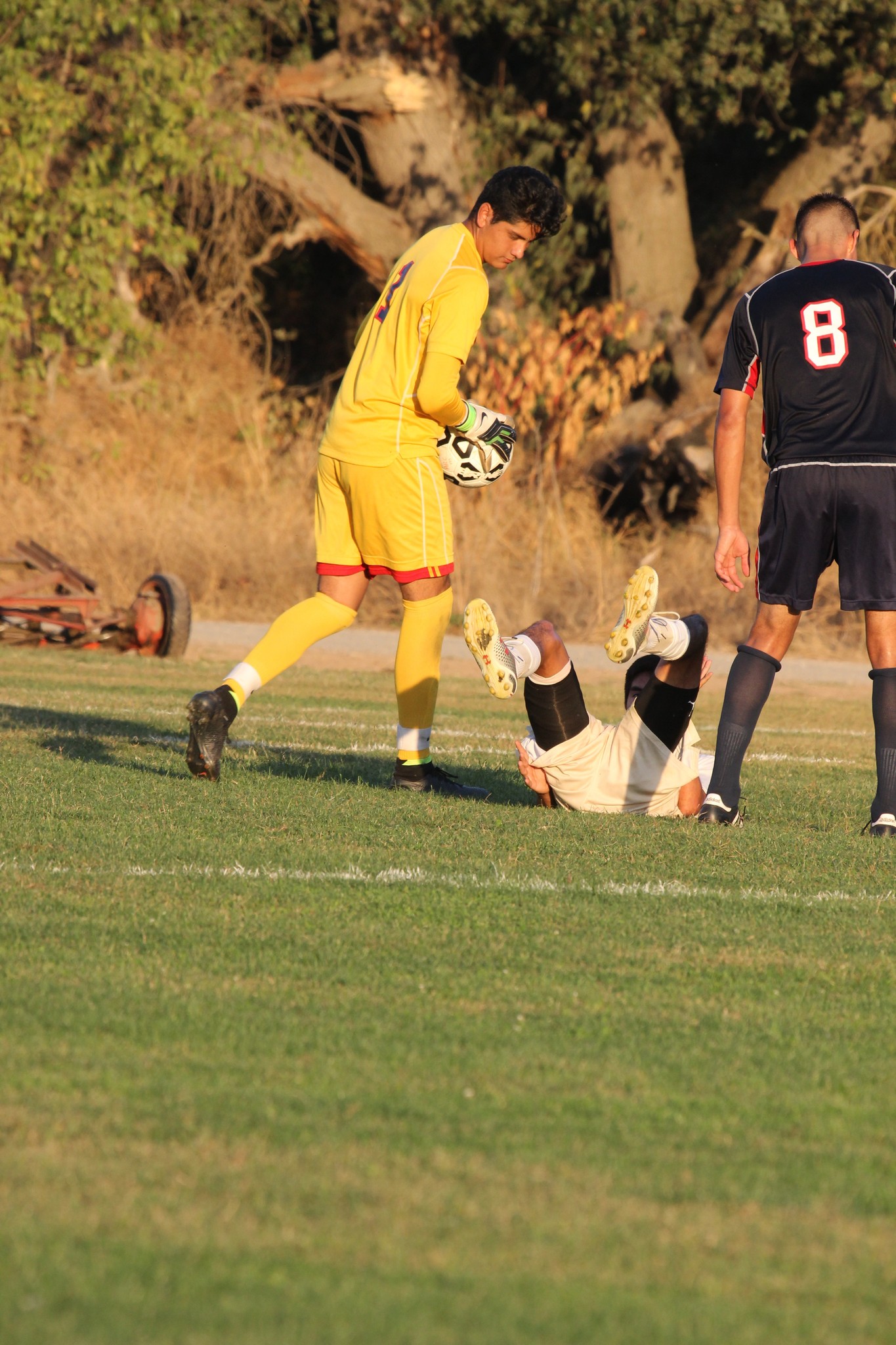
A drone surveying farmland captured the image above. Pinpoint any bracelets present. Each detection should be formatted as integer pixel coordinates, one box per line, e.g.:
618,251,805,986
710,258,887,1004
453,398,478,433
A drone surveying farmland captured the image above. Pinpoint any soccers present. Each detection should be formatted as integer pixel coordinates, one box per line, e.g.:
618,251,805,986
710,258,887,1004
440,405,514,487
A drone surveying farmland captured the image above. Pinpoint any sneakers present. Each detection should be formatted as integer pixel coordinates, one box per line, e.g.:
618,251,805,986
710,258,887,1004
867,806,896,840
461,597,518,699
182,690,222,784
388,760,492,804
604,565,659,664
696,791,745,830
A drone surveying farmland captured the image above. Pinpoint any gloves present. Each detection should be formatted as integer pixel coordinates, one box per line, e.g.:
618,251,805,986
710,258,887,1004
453,398,518,473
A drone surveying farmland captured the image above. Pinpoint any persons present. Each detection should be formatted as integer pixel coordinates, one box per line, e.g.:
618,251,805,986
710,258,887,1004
461,565,743,816
185,165,571,799
698,192,896,837
